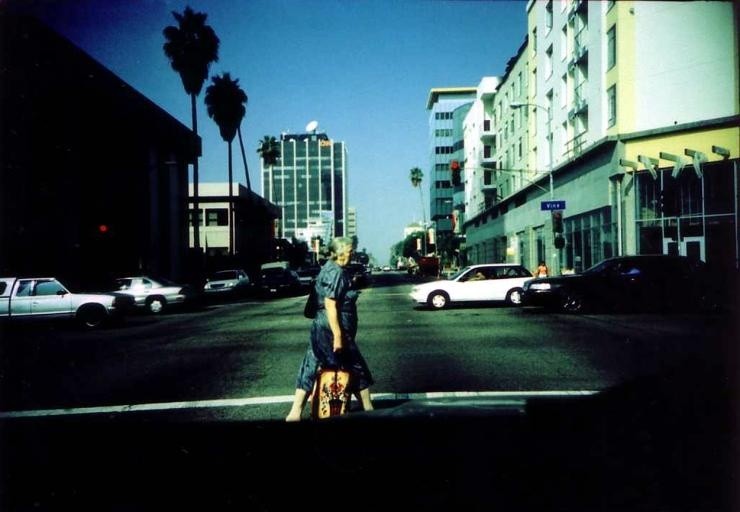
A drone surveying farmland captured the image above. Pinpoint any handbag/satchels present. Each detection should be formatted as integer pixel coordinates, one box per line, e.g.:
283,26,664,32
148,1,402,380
307,363,354,420
301,277,319,320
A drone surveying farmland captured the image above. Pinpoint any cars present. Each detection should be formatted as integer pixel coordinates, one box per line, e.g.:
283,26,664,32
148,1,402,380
0,277,191,330
373,266,391,271
346,264,371,290
203,260,320,295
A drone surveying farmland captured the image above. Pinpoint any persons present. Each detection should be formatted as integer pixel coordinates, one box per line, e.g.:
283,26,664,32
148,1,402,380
283,235,377,422
534,261,550,279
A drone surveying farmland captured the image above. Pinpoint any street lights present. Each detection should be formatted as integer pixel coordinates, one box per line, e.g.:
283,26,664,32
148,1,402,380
451,161,460,187
509,102,561,276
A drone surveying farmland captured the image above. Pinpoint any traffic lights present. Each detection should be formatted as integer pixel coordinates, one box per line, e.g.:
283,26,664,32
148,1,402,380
552,210,565,249
